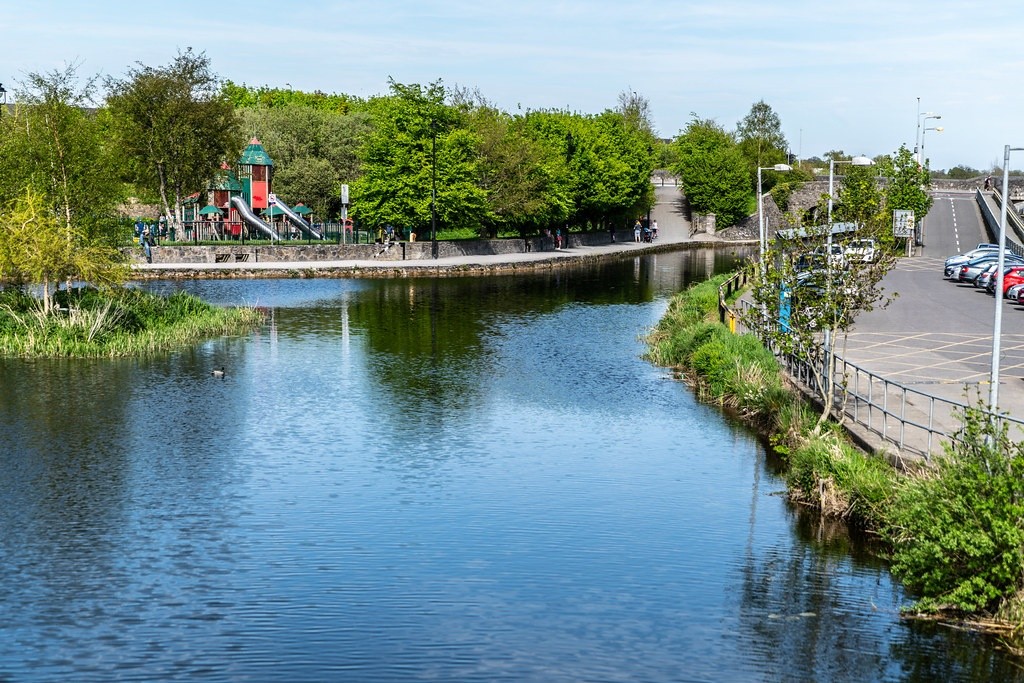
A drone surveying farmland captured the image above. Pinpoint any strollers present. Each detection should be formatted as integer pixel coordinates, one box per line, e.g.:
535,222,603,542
642,228,652,243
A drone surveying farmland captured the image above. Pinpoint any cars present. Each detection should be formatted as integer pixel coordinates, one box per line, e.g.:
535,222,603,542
789,238,880,326
944,243,1024,304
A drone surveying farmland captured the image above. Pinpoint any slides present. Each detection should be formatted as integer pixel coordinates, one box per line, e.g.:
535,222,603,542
231,194,284,241
268,198,326,241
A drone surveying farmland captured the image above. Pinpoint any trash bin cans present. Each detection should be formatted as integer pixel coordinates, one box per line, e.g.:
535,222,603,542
914,242,923,256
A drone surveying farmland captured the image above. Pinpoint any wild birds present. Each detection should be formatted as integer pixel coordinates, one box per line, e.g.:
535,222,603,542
211,367,225,374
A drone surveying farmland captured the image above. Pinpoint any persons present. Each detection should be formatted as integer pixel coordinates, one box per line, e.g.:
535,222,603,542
555,227,562,249
608,221,616,244
527,238,533,252
652,219,659,239
134,220,157,262
633,220,643,243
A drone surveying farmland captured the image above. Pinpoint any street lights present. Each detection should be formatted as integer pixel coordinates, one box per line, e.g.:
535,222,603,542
756,163,793,345
429,117,441,259
821,156,877,394
915,110,943,244
